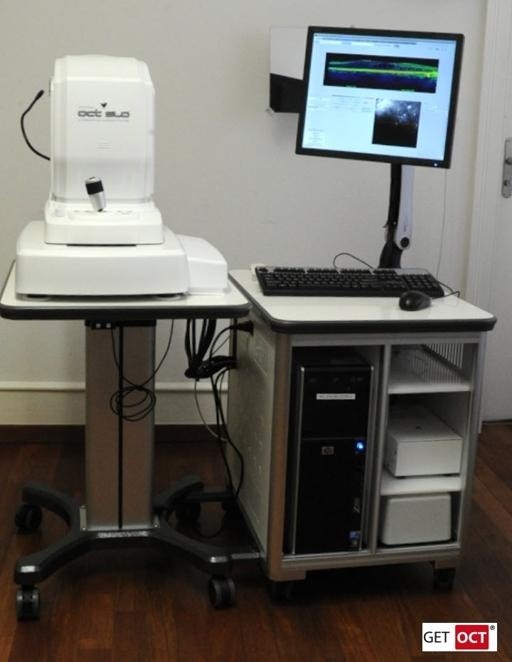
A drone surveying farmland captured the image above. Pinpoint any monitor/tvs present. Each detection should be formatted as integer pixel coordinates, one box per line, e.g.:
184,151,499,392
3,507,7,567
295,26,465,169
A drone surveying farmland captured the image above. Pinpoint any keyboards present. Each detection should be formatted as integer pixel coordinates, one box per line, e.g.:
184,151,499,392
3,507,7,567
255,266,444,297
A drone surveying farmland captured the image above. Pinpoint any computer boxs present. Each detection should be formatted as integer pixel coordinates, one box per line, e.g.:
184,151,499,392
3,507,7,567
283,347,380,555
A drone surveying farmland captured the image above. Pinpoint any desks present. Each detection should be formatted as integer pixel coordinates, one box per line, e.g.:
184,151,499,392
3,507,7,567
0,231,251,626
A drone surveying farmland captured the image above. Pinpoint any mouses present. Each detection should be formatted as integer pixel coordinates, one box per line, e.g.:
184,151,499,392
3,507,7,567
399,291,431,309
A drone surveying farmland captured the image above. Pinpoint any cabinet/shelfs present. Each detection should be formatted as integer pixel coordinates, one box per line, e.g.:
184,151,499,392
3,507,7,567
227,265,497,604
